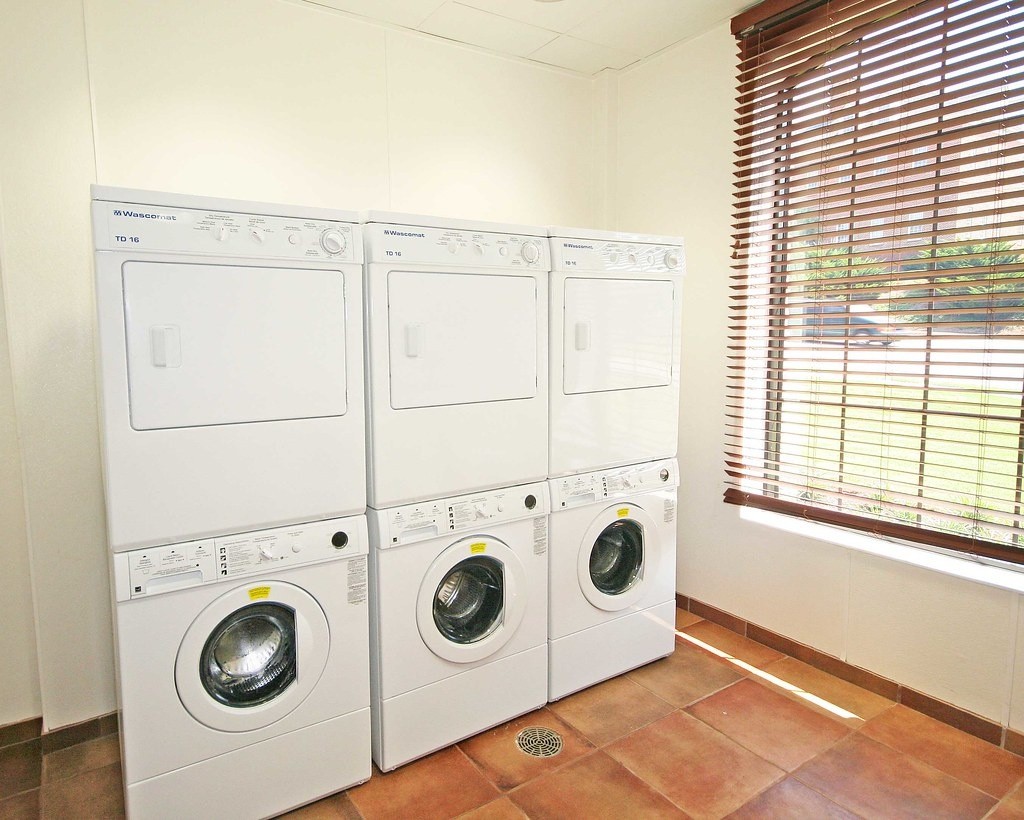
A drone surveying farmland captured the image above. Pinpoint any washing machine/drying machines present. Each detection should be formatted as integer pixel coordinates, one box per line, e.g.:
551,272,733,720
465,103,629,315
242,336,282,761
86,179,685,819
